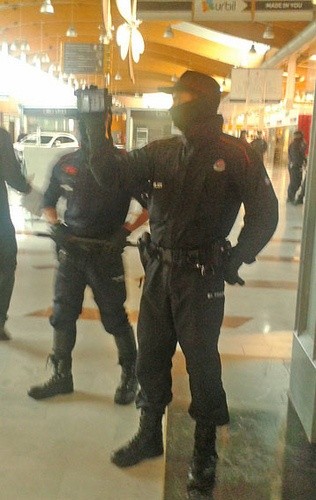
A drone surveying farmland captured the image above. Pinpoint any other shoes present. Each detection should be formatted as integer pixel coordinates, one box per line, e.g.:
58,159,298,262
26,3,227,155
293,199,303,205
287,194,295,202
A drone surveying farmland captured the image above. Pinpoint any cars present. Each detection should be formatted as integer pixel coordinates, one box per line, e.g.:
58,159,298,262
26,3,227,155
13,131,79,162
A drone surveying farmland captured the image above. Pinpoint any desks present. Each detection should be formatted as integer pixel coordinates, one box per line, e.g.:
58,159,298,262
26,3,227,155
22,146,81,222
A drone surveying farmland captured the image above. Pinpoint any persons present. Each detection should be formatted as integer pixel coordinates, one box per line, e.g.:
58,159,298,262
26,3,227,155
295,173,307,204
287,130,306,202
239,129,250,141
26,84,150,405
250,130,269,162
0,126,44,342
82,70,280,500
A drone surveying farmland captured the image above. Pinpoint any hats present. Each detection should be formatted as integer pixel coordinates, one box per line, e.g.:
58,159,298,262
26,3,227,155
158,71,220,100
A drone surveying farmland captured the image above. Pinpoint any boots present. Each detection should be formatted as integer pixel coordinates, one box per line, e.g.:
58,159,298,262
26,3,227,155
26,353,74,400
187,423,219,500
114,366,138,405
111,408,165,469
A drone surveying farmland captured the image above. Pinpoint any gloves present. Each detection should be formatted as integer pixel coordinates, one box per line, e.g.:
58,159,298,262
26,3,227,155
23,189,46,218
219,247,244,285
101,227,131,264
53,224,73,253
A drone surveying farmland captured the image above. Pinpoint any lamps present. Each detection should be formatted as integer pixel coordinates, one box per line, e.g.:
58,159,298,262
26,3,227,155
162,23,173,38
261,22,275,40
40,0,55,14
65,25,78,37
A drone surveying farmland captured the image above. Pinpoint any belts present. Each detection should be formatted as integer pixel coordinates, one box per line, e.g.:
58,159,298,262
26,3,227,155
72,235,103,256
150,241,225,268
